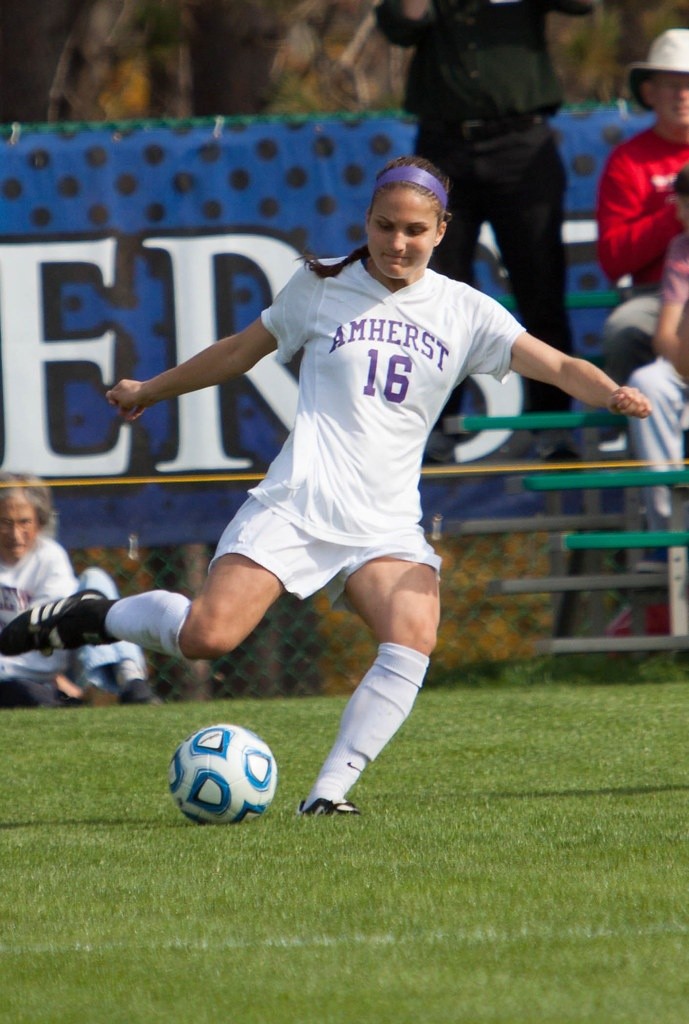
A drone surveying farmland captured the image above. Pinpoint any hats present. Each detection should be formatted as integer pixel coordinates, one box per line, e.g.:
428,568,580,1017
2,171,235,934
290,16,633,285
627,28,689,113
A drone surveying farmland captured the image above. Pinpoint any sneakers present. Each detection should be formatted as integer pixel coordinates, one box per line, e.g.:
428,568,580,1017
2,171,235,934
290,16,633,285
0,588,108,656
297,799,361,816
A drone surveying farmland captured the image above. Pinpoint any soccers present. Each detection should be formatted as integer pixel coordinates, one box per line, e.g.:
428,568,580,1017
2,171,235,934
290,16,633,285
168,724,279,827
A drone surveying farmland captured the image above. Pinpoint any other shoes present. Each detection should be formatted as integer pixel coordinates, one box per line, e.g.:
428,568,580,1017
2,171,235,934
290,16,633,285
54,672,93,705
425,440,464,465
120,678,158,705
533,434,580,460
634,547,669,573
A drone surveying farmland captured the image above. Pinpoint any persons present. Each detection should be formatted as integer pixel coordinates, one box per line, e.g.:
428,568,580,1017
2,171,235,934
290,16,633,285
604,162,689,573
0,158,653,816
373,0,594,467
151,542,284,697
0,471,163,709
583,29,689,387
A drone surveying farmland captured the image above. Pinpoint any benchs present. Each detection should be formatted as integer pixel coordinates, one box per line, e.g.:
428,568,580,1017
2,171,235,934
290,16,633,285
447,291,688,660
